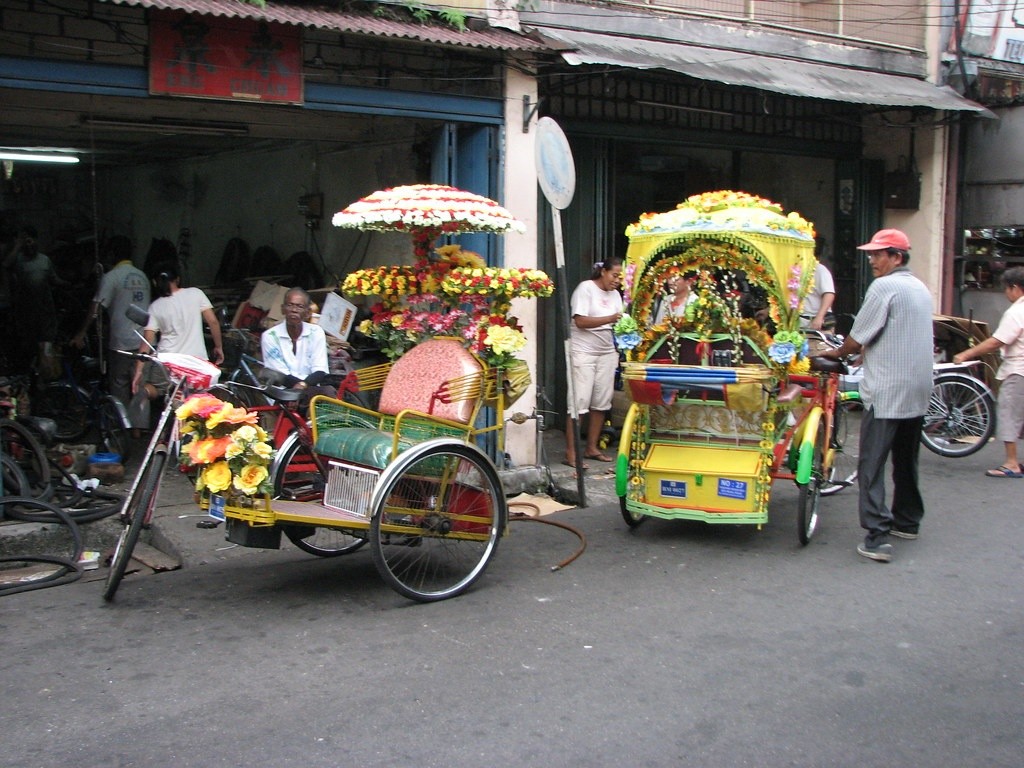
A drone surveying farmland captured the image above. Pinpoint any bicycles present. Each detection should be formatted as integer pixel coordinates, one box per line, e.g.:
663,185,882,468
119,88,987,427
32,339,133,465
920,360,998,458
221,326,373,436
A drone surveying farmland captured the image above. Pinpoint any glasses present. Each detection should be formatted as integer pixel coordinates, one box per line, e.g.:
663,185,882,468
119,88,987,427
868,252,889,261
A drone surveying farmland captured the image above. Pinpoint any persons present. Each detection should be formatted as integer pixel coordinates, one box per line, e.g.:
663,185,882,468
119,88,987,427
0,225,78,359
74,236,152,437
132,261,224,397
260,288,331,417
953,266,1024,478
653,267,700,326
799,235,836,338
817,228,934,562
562,256,625,468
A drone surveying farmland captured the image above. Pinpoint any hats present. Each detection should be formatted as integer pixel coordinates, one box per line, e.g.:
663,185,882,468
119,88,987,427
856,228,911,251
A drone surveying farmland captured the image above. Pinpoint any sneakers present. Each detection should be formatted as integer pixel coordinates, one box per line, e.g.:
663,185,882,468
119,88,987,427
889,526,919,539
856,542,893,562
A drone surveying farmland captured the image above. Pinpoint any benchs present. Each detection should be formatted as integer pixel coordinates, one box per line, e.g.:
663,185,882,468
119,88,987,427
619,361,818,446
310,338,493,513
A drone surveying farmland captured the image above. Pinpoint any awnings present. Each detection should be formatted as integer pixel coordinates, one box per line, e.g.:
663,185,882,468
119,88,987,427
94,0,576,53
526,23,1000,121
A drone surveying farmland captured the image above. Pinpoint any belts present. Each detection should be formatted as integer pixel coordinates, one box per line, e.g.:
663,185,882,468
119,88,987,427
800,315,815,320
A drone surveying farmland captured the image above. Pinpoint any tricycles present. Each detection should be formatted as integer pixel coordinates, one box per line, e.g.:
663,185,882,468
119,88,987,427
610,188,862,547
98,183,556,607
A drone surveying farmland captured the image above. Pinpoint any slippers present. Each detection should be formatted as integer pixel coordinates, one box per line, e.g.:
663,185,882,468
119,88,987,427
561,460,590,468
985,466,1024,478
583,452,613,462
